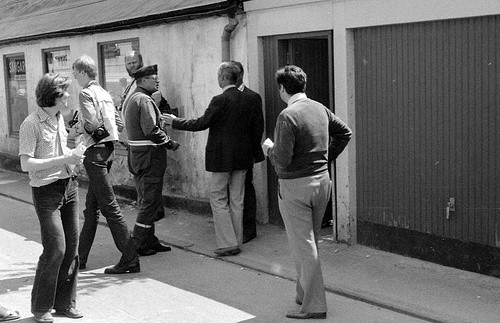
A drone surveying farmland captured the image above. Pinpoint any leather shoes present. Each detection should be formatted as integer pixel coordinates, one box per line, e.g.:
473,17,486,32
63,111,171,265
80,264,85,269
105,256,140,274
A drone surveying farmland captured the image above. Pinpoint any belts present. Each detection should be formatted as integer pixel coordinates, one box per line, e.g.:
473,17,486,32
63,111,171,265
88,141,112,148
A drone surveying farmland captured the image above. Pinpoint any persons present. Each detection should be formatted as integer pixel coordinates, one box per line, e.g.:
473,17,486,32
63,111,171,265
0,303,21,322
229,60,263,244
161,62,263,255
127,64,180,256
262,64,352,320
119,50,167,221
72,56,139,275
18,73,85,323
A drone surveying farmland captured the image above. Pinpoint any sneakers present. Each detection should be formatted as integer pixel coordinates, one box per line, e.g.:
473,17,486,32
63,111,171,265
214,246,241,254
56,307,83,318
0,305,21,320
34,311,54,322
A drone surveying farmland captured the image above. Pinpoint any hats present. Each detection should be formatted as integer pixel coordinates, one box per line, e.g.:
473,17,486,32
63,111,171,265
133,64,158,80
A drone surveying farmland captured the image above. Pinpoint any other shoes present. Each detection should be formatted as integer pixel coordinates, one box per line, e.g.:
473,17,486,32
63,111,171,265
286,310,326,319
153,243,171,251
296,298,302,305
243,232,257,243
139,246,156,255
